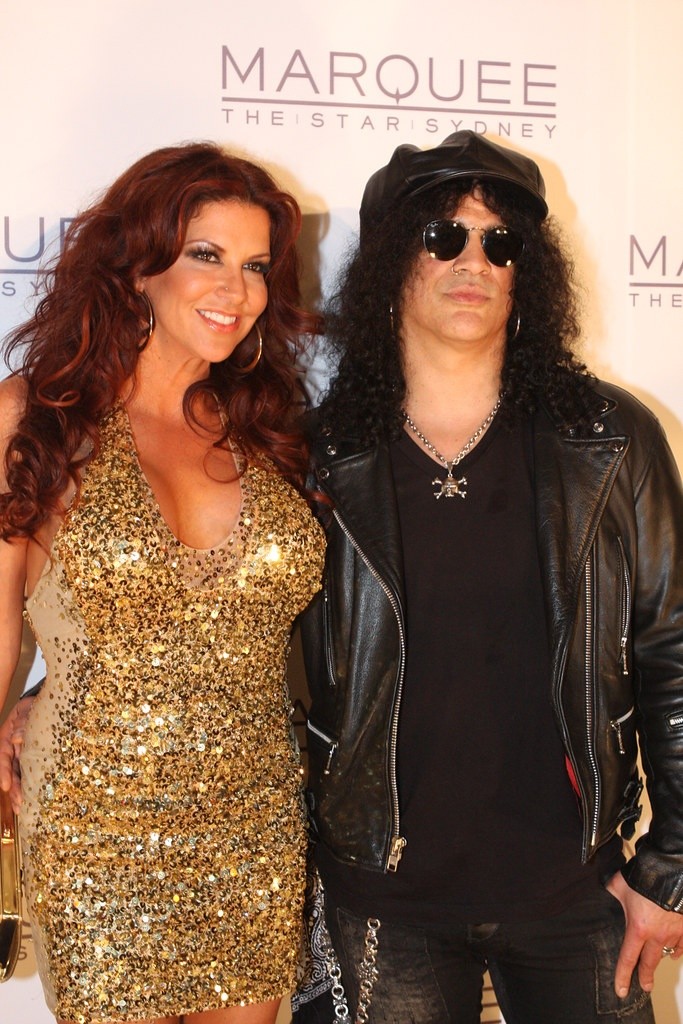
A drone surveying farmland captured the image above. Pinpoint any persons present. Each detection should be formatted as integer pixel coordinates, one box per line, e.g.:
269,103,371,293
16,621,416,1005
0,132,683,1024
2,144,327,1024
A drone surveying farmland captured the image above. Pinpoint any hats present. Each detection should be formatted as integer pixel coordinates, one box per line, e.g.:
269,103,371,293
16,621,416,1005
360,131,548,227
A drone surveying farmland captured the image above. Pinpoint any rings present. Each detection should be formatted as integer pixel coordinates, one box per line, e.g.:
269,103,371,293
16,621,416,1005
663,947,677,953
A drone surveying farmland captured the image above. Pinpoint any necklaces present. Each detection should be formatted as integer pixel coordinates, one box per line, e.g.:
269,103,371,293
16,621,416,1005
402,393,502,498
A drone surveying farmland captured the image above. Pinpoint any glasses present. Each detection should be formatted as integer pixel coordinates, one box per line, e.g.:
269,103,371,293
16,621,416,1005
402,218,522,267
